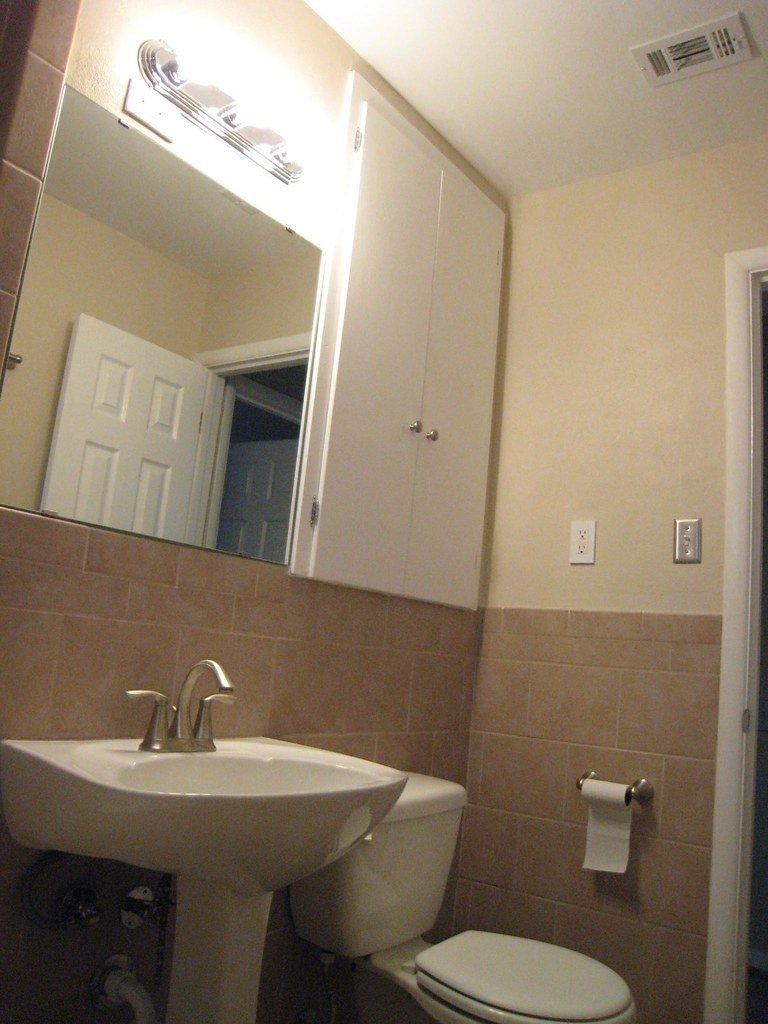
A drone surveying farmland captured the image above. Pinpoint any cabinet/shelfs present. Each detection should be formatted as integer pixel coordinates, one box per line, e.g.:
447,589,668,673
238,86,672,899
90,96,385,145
307,101,504,611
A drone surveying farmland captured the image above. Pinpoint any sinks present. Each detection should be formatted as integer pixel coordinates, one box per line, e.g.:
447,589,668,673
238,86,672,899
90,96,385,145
0,731,410,891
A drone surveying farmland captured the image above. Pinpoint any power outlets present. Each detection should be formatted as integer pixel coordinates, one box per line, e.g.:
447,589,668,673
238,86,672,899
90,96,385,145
568,519,596,564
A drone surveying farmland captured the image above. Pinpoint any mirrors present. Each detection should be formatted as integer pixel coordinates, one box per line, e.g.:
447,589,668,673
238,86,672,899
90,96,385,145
1,84,322,567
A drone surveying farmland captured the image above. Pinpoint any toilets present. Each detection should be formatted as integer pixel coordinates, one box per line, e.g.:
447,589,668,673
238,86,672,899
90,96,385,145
290,772,637,1023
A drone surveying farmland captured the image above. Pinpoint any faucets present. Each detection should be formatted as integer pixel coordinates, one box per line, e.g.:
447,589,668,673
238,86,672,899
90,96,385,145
168,658,236,753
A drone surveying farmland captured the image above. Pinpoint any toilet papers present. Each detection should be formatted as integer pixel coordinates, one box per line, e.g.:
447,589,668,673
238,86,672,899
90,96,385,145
580,777,633,875
